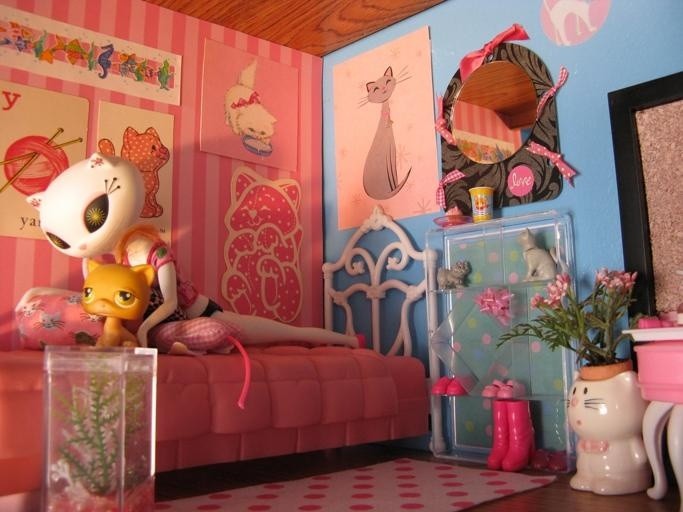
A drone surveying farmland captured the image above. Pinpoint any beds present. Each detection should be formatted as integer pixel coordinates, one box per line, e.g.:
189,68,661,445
2,202,445,512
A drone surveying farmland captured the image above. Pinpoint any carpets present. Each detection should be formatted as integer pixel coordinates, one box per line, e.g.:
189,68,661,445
155,454,556,512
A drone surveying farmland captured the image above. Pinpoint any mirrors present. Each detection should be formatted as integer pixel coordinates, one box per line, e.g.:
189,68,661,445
436,40,563,210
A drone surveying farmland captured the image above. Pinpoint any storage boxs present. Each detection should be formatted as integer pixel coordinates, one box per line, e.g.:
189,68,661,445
633,345,683,404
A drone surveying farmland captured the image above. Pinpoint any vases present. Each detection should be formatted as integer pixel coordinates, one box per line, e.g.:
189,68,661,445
567,365,650,494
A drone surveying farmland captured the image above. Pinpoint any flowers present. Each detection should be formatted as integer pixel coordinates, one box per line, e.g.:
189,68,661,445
493,265,649,365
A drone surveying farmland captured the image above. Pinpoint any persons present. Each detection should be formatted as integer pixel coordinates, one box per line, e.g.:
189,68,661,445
26,151,367,348
